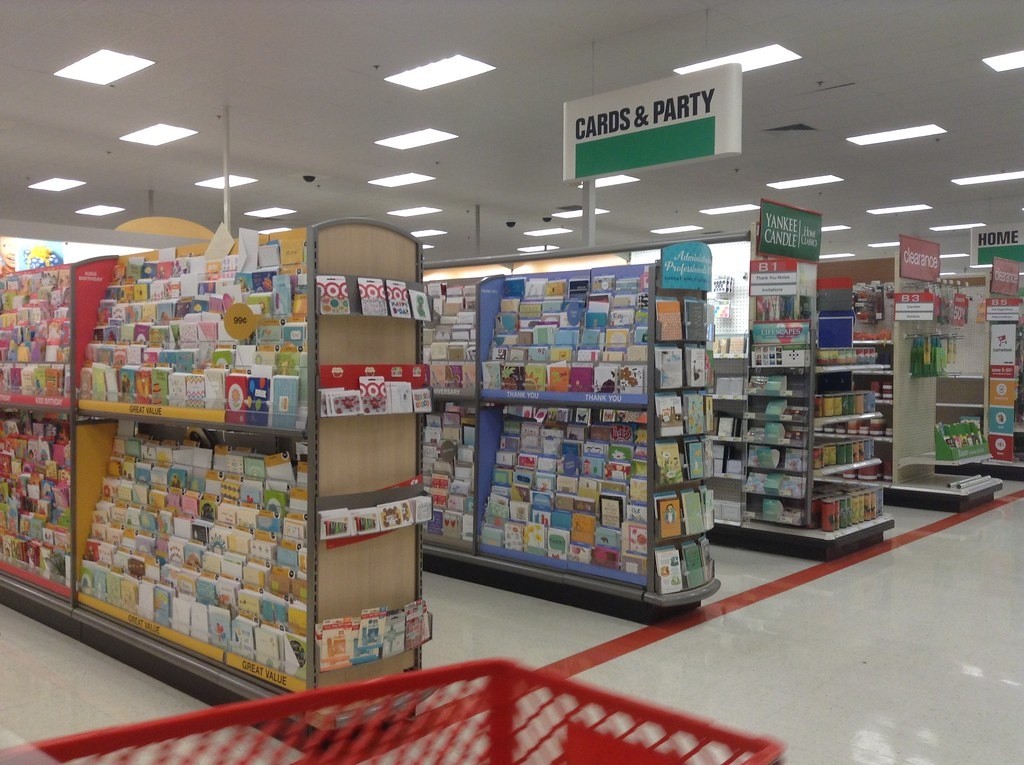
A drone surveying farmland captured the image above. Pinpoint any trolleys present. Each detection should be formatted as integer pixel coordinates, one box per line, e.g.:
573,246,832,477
1,658,790,765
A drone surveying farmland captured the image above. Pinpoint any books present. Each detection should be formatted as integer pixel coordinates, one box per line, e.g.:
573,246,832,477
2,224,306,672
420,262,714,594
713,273,747,521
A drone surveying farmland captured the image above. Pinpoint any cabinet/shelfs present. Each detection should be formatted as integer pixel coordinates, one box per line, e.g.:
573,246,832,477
0,197,1024,765
